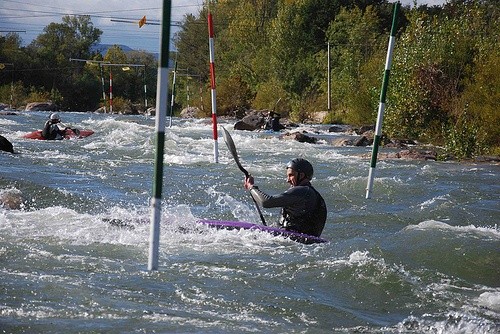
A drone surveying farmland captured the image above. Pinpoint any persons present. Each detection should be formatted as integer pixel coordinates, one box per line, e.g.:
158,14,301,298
41,113,71,140
244,157,327,237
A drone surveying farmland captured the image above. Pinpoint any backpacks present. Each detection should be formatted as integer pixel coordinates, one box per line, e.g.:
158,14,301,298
41,121,54,138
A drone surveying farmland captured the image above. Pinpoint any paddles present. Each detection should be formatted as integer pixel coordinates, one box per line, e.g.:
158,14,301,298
60,121,81,135
220,124,266,226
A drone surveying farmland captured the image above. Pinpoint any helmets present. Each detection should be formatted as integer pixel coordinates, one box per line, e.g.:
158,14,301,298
287,157,314,181
50,113,60,119
